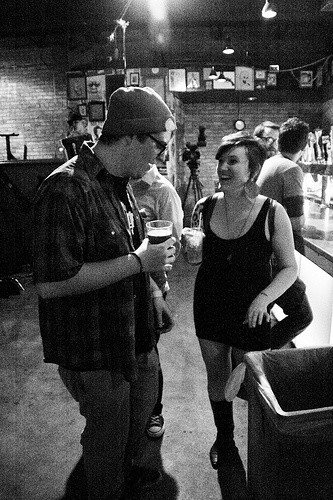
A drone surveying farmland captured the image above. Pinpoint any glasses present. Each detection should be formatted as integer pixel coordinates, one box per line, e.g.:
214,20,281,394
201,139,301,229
147,134,168,158
260,136,278,146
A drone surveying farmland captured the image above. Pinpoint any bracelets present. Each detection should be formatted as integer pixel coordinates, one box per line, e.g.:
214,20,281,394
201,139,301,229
127,252,143,274
259,292,269,298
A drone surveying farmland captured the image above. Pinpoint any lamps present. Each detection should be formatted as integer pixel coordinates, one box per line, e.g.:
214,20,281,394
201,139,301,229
261,0,277,19
222,35,235,55
217,68,226,82
209,66,217,77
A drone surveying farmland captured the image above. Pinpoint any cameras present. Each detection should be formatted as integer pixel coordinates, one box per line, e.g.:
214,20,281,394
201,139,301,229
181,143,201,175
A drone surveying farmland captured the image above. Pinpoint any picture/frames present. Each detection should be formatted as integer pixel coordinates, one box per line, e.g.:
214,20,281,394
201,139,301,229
77,104,88,117
67,72,89,101
88,101,105,122
169,64,313,92
130,72,139,87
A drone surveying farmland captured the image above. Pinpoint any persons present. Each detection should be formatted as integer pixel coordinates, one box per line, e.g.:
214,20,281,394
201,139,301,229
128,160,185,438
29,86,178,500
253,120,280,159
255,116,310,230
179,131,299,466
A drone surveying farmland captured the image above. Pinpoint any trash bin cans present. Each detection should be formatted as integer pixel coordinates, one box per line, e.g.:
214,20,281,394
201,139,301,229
243,345,333,492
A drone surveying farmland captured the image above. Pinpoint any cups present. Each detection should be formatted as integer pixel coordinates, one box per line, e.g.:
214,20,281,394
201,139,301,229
187,227,204,266
146,219,176,246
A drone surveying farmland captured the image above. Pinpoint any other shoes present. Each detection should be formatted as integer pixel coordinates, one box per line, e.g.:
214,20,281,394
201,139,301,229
125,465,162,490
210,436,235,469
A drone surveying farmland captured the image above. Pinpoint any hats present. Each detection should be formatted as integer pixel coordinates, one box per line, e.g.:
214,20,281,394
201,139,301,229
101,87,177,135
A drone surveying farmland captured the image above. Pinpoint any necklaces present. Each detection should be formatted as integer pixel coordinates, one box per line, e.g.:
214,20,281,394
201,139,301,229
223,193,256,262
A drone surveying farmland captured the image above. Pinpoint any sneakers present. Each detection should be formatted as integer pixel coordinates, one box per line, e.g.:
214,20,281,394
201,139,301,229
147,413,165,438
